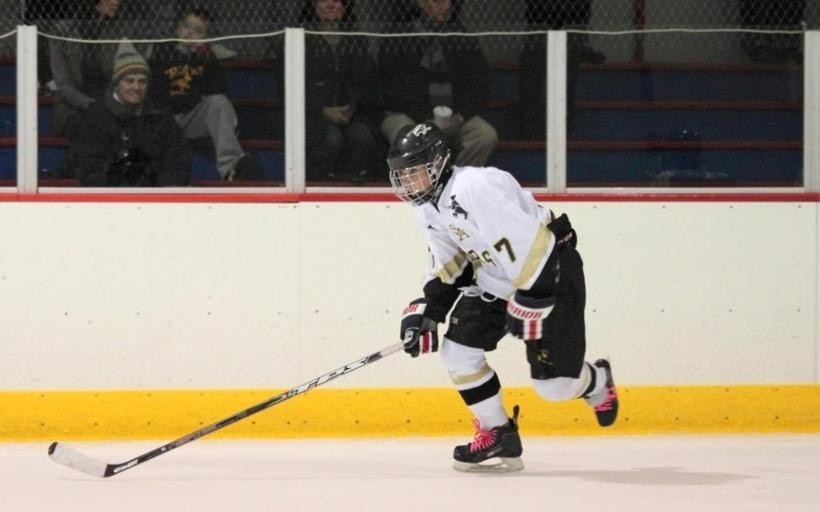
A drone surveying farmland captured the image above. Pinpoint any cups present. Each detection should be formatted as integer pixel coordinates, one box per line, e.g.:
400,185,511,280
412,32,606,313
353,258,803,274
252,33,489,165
432,105,454,130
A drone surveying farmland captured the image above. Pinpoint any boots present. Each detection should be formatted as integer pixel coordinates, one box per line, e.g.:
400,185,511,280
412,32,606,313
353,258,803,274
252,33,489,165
454,405,523,463
585,359,618,427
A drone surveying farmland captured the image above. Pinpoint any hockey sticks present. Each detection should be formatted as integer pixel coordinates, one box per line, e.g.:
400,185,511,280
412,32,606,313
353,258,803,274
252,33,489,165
48,338,411,478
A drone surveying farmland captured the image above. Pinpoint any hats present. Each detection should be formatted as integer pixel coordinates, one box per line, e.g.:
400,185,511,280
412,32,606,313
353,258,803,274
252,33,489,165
111,35,151,88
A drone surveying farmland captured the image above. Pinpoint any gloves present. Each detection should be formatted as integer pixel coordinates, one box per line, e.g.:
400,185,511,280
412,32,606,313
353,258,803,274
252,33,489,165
399,299,438,359
504,291,556,343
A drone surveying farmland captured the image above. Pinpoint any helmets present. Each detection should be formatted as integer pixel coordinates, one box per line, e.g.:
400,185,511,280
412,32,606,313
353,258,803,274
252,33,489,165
389,121,452,208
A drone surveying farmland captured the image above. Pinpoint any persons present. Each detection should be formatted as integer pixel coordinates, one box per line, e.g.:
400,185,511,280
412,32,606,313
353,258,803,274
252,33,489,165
29,1,498,187
519,1,608,66
388,123,619,464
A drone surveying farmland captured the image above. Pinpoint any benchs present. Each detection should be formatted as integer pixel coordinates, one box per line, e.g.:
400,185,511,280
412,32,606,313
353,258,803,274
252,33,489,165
0,50,803,187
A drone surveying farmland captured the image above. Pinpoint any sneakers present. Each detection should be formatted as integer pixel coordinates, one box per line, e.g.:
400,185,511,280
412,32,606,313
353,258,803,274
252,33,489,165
224,153,252,181
